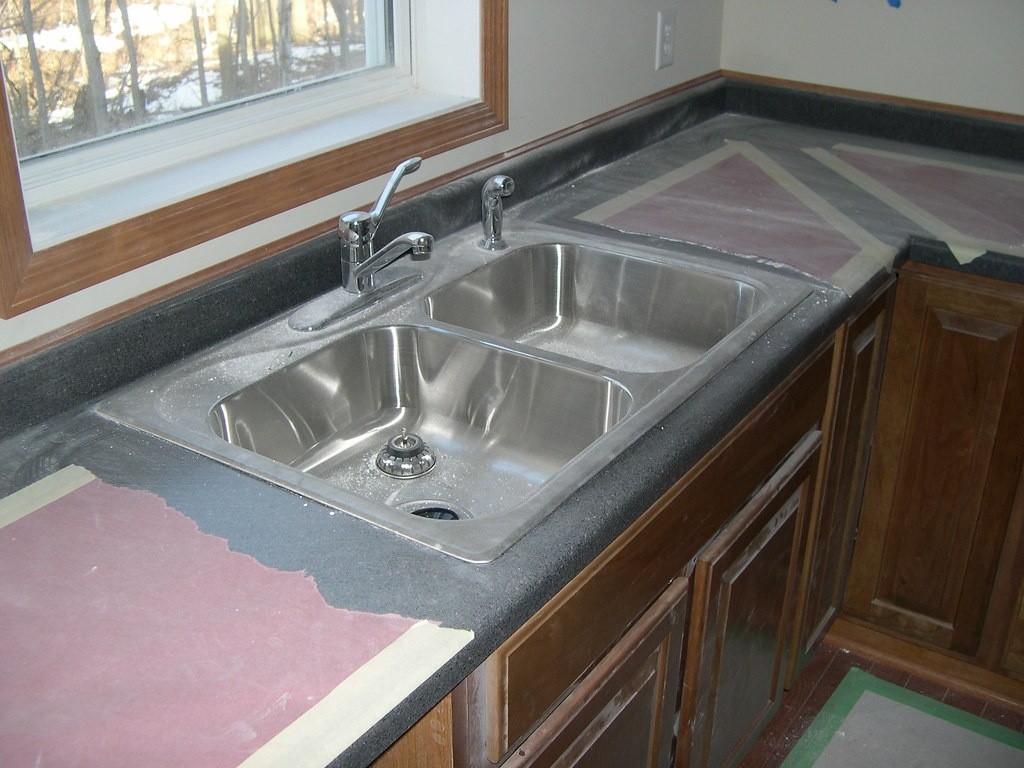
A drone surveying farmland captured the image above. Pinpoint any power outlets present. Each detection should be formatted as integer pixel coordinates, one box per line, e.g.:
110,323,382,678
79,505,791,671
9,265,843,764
655,8,675,70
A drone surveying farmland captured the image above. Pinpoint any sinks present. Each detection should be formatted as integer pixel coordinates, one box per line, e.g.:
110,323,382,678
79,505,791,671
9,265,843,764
349,210,814,390
90,285,698,566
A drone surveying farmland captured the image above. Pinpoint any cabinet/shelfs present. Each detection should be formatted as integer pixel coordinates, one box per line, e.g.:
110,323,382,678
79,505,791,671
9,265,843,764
367,261,1024,768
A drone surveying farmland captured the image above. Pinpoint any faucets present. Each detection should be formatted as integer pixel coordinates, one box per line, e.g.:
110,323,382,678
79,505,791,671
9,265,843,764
338,155,435,294
477,174,516,251
